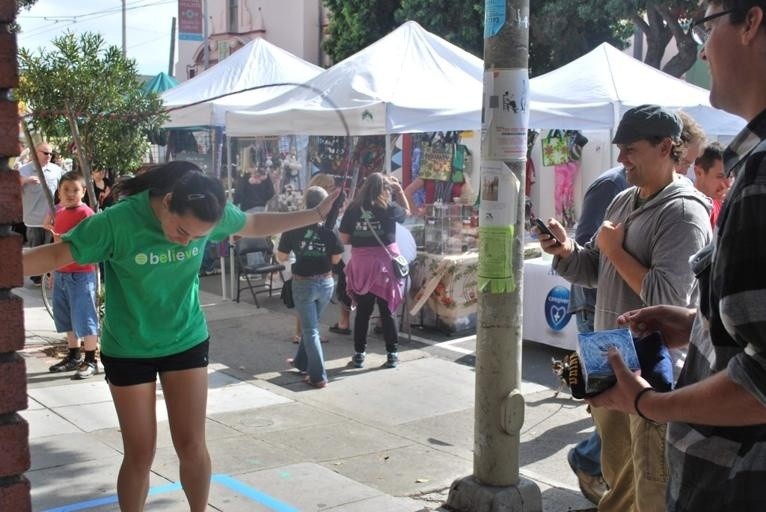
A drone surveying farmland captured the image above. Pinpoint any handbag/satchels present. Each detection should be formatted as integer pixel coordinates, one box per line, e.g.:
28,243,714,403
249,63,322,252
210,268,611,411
417,133,454,184
446,132,464,184
540,130,570,167
464,145,472,175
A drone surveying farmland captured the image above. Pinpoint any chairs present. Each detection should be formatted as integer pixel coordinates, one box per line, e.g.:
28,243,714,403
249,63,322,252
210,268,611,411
231,235,284,307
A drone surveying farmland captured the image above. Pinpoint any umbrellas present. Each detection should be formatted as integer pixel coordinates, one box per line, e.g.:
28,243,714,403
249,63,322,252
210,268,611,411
222,18,484,138
528,40,752,141
53,71,214,134
145,36,328,131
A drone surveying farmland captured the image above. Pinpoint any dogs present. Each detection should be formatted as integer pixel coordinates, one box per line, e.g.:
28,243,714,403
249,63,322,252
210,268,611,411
550,355,571,397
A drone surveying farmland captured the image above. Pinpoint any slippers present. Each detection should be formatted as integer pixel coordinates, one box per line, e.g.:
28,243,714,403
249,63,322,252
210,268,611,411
327,321,353,336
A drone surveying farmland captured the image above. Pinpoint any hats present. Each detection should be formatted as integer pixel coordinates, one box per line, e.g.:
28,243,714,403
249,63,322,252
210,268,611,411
610,103,685,146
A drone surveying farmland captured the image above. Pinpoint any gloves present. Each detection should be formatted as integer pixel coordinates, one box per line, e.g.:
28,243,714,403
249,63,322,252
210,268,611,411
568,131,589,162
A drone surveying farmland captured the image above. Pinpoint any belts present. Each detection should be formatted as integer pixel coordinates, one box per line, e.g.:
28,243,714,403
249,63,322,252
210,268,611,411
291,270,335,283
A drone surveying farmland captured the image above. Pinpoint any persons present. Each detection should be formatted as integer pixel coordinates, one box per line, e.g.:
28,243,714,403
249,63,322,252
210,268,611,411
694,139,735,232
529,103,719,503
276,184,344,390
337,172,412,368
565,107,709,512
21,156,343,512
582,0,766,512
233,169,280,283
329,198,354,335
43,177,65,288
294,171,330,345
198,240,223,276
82,162,114,283
48,169,101,380
21,141,66,288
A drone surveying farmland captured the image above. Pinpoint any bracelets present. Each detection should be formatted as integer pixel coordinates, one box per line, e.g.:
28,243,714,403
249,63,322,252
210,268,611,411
312,202,327,225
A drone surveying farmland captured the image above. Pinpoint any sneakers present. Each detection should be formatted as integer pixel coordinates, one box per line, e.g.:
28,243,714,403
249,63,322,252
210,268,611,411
384,352,399,369
304,374,329,391
292,333,302,344
48,354,86,374
284,357,305,371
567,446,609,504
319,335,329,343
351,352,366,370
73,360,99,379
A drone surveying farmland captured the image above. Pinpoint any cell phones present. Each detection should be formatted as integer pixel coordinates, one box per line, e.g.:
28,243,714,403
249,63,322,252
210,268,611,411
530,217,561,247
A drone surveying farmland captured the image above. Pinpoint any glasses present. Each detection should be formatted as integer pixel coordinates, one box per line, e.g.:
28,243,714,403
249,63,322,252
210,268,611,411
687,7,747,46
35,151,54,156
